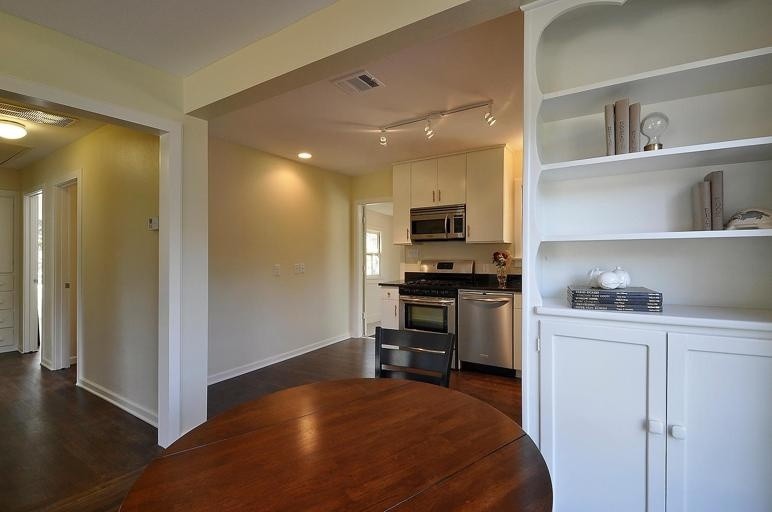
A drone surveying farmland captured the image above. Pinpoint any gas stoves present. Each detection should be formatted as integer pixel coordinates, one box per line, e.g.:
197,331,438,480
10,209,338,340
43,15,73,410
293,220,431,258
399,278,469,298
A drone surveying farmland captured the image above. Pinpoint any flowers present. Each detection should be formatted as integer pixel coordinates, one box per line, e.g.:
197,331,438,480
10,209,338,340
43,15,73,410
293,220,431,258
492,248,513,281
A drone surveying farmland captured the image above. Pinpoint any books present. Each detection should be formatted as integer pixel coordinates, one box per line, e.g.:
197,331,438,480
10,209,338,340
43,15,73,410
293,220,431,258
604,98,640,155
566,285,662,312
703,171,723,231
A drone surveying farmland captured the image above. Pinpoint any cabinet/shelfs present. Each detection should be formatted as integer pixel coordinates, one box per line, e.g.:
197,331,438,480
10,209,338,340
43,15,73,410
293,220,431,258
380,286,399,350
409,151,467,208
390,158,413,247
519,0,770,511
465,143,515,245
0,189,18,356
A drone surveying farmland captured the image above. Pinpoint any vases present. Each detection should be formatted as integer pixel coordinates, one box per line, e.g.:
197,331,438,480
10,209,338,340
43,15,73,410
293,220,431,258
495,264,509,290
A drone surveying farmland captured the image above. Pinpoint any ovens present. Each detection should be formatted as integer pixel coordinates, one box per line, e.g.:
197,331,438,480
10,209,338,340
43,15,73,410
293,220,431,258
397,296,455,369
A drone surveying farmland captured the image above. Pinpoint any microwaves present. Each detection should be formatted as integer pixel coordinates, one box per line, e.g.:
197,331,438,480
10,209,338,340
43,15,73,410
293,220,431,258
410,205,465,241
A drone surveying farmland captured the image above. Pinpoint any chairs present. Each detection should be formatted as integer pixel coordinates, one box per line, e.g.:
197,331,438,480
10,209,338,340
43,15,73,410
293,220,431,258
373,324,455,391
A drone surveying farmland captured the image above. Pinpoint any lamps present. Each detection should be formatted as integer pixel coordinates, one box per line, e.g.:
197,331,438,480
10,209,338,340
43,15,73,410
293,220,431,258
377,110,497,148
0,118,28,141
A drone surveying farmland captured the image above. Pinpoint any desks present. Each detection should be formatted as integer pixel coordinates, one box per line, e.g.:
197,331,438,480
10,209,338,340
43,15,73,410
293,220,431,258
120,375,554,509
